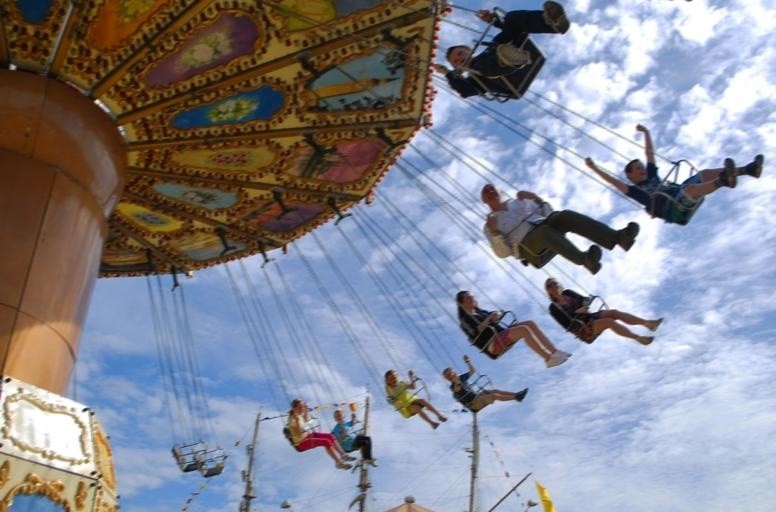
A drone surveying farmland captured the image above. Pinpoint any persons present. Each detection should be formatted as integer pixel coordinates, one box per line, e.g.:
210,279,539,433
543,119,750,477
443,354,529,412
585,123,763,225
482,183,640,275
384,369,448,429
288,398,357,470
545,277,664,345
434,0,570,98
456,290,573,368
331,408,378,468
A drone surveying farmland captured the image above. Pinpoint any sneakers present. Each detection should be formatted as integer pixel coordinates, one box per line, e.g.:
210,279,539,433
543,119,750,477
546,350,571,368
516,388,527,401
617,222,640,251
583,245,602,274
719,158,736,188
336,454,356,469
745,154,762,177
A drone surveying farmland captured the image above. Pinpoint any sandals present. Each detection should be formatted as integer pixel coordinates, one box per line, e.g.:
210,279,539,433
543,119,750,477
637,337,652,345
645,318,662,332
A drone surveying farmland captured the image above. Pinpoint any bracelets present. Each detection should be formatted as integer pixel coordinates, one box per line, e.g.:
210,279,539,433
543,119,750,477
491,231,497,236
465,360,472,365
